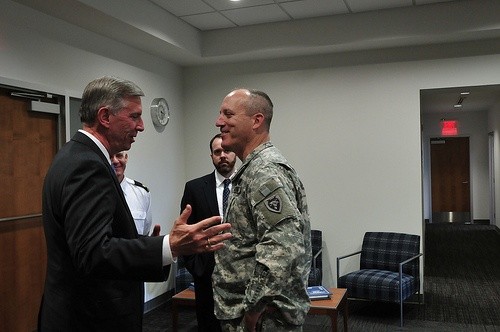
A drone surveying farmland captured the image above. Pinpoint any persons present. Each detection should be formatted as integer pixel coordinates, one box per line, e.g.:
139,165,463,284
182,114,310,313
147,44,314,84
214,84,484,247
212,89,313,332
112,151,152,236
179,134,240,332
37,78,232,332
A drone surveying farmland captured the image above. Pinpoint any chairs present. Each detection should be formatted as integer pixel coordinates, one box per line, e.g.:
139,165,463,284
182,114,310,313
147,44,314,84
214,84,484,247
308,230,324,286
336,232,423,328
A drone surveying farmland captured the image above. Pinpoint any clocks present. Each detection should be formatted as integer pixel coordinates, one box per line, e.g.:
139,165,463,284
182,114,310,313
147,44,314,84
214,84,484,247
150,97,171,127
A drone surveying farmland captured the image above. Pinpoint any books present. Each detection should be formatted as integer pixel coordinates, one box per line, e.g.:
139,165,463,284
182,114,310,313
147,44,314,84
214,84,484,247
307,284,333,301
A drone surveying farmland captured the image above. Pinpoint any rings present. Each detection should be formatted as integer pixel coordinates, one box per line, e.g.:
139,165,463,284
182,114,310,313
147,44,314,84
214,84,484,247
207,239,210,245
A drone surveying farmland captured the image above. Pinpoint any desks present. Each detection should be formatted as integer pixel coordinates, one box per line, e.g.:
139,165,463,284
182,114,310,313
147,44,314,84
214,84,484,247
172,287,348,332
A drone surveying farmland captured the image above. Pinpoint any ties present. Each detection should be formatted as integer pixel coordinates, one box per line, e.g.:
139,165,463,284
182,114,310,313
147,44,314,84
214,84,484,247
111,162,117,175
222,179,231,215
